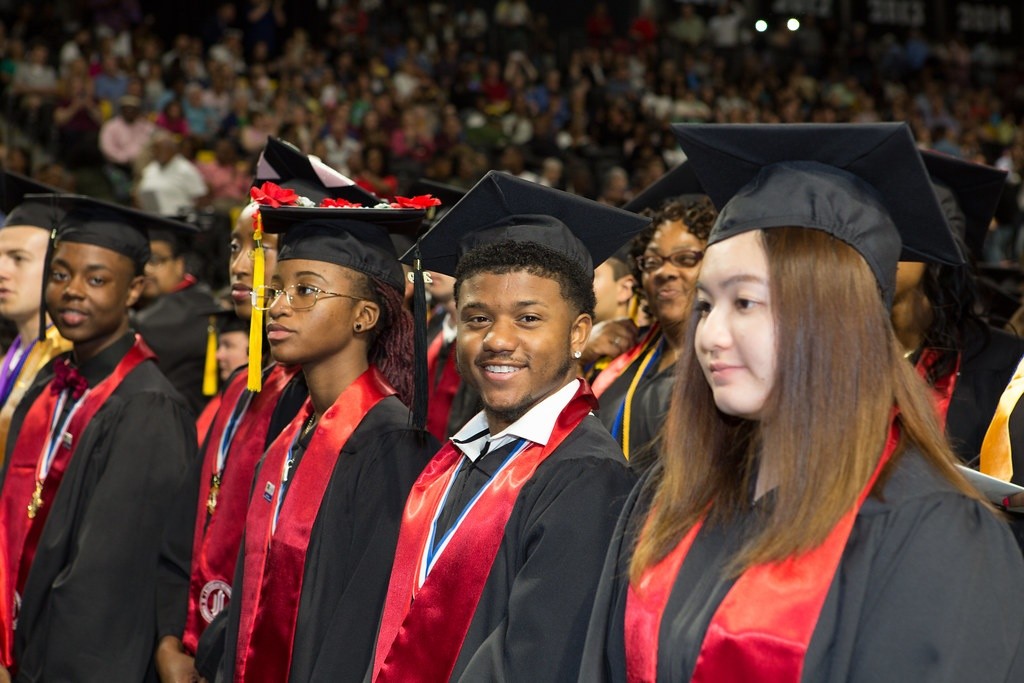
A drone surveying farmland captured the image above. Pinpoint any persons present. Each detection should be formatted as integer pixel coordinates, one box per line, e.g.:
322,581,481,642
2,0,1024,507
147,186,356,683
540,112,1021,683
200,201,444,683
0,175,206,680
338,161,645,683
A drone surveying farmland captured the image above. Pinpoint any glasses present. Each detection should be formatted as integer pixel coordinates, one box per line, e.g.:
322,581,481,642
636,249,703,272
251,285,367,310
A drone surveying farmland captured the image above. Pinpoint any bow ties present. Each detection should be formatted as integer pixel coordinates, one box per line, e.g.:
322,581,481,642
51,362,88,399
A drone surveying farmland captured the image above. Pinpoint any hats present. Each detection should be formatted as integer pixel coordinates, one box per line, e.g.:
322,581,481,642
673,124,963,312
624,157,708,213
397,169,654,277
1,168,71,233
147,214,193,253
250,134,392,211
915,146,1006,264
23,191,197,263
253,206,424,266
